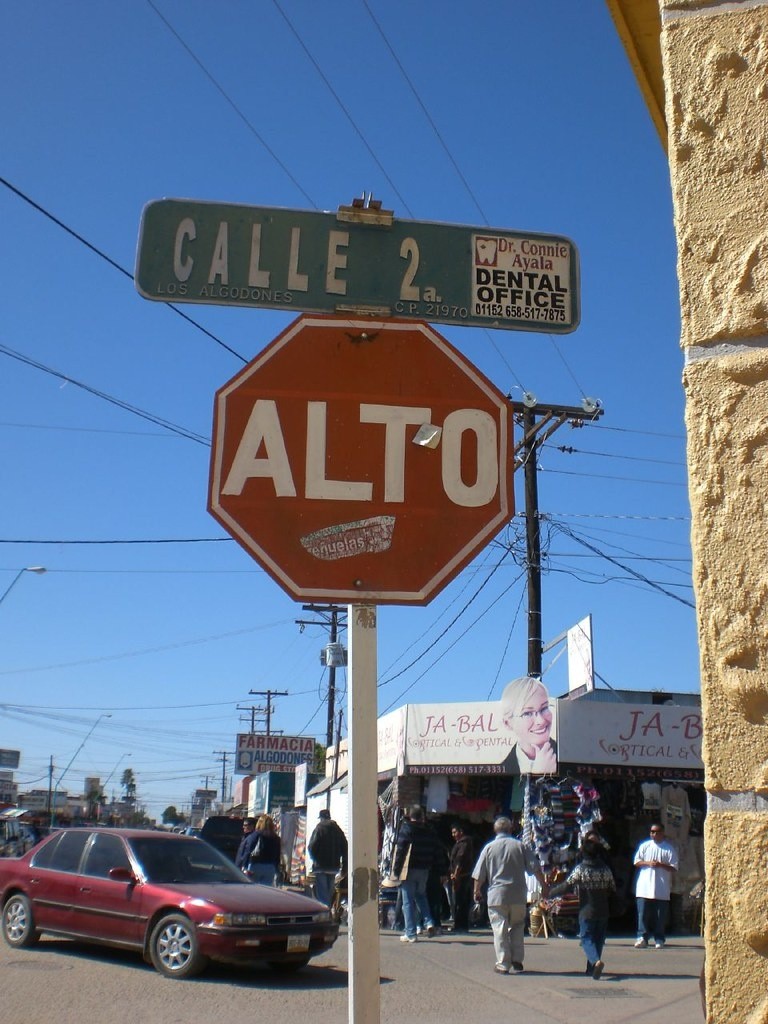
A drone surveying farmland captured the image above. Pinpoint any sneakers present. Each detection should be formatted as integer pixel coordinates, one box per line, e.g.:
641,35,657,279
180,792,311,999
635,937,647,948
655,939,663,948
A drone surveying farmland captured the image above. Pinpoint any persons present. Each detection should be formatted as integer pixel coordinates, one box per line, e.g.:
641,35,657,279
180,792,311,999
448,822,473,932
390,802,436,944
235,817,257,872
242,815,281,886
151,826,158,831
549,831,616,981
499,676,556,774
307,809,349,905
633,820,680,952
471,815,551,975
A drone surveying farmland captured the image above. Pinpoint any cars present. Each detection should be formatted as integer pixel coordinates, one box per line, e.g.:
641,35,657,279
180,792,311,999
0,827,340,979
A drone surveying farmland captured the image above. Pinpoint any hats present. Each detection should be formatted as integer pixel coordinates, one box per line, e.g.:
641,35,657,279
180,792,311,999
318,809,330,819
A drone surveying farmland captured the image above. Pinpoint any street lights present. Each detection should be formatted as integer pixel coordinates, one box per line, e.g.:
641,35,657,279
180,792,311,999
52,714,111,825
89,753,131,820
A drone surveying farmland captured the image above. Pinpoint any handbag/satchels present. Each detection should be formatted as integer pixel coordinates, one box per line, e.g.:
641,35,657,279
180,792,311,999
248,834,264,862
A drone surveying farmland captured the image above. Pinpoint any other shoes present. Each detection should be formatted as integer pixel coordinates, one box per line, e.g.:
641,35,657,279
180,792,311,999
592,960,605,980
416,927,423,935
426,925,435,938
512,961,524,973
494,962,510,974
447,926,469,933
400,934,417,942
584,961,593,975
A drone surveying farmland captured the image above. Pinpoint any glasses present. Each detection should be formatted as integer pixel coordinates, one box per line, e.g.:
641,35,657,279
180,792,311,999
509,705,554,721
650,830,663,835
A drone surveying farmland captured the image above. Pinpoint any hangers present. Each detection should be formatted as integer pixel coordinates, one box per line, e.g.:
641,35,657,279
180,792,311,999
647,779,701,787
517,770,583,789
